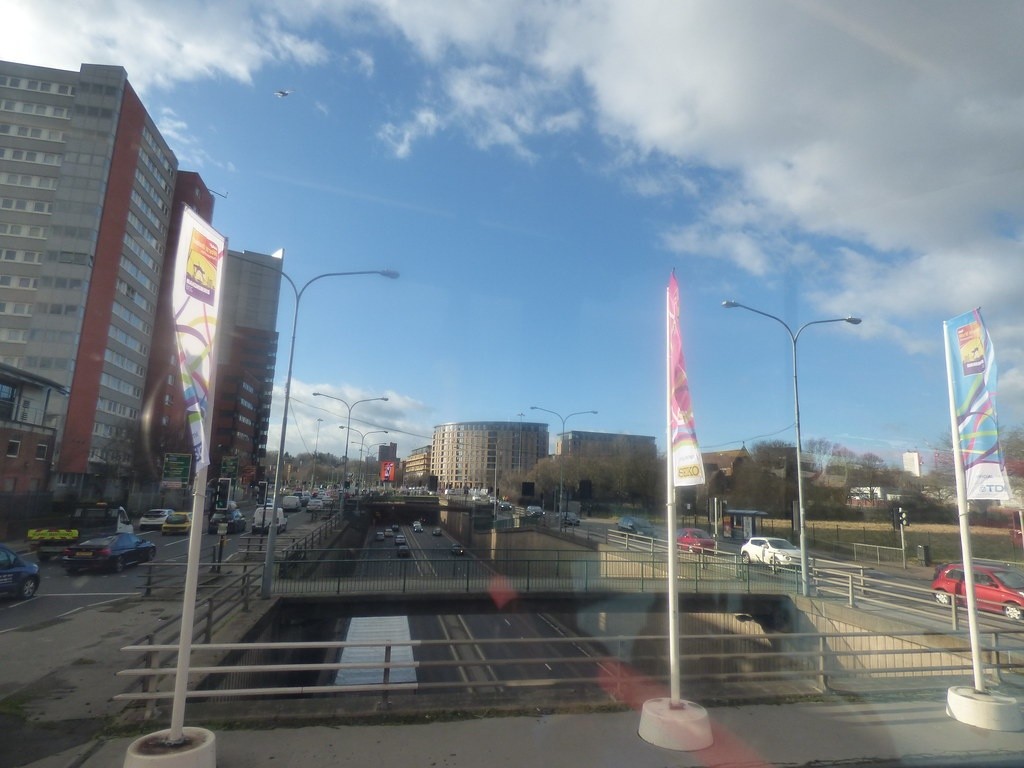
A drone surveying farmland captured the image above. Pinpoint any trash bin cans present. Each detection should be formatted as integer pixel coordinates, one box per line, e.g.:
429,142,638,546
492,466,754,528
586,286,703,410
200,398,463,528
917,544,930,566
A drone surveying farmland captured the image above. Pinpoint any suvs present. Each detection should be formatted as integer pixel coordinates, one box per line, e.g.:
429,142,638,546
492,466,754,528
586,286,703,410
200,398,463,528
617,516,657,540
525,506,546,518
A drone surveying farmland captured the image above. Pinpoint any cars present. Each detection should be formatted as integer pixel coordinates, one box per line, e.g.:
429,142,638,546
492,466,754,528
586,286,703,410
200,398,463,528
208,507,246,534
376,520,410,558
676,528,716,552
0,546,41,600
432,526,442,536
413,521,424,533
741,537,801,568
472,494,513,511
450,543,464,555
306,488,334,512
562,511,580,525
930,557,1024,619
60,531,158,576
139,509,194,536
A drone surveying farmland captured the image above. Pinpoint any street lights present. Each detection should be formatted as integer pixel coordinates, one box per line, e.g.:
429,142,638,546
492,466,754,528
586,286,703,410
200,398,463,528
530,406,599,532
720,300,863,596
312,392,389,525
227,253,399,600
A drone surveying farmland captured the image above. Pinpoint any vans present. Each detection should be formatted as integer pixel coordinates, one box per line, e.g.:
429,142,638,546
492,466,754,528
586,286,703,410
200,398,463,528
282,486,310,511
252,507,288,535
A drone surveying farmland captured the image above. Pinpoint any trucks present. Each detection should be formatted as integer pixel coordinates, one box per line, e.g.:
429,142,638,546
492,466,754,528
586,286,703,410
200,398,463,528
27,500,135,562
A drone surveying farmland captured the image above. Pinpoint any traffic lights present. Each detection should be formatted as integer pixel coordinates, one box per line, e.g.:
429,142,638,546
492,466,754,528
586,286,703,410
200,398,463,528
256,482,266,505
215,479,231,509
